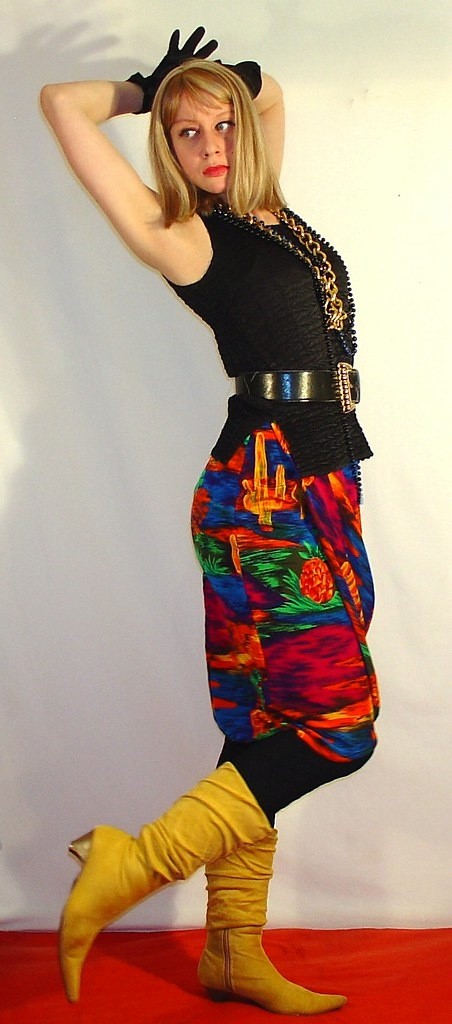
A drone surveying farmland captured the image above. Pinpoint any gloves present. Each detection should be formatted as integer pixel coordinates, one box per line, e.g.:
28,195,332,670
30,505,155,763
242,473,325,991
213,59,262,101
124,26,218,114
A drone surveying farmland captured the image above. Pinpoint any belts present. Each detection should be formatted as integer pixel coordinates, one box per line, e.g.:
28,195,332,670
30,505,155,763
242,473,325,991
234,362,361,415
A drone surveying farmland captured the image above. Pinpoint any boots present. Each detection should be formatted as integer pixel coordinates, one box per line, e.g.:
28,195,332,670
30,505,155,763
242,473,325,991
197,827,346,1016
57,761,274,1003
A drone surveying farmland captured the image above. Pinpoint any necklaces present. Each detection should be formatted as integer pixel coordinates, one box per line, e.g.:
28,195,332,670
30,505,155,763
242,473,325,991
208,203,362,505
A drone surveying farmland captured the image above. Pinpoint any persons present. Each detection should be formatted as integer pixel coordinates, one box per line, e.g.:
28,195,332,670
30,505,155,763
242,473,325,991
41,26,379,1014
